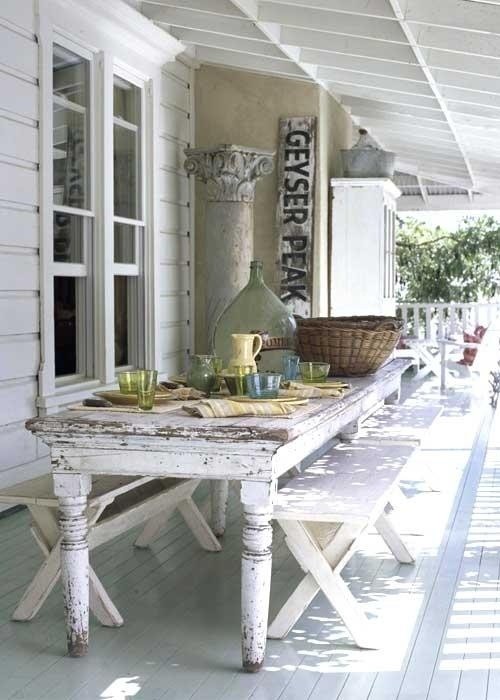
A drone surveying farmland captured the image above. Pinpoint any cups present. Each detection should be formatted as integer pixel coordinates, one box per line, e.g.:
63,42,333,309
297,361,332,385
230,364,254,397
209,357,224,393
117,370,139,395
280,354,301,381
244,372,283,399
136,368,159,411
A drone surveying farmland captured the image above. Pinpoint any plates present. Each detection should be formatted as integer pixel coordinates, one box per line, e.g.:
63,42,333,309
286,378,350,388
91,387,178,407
220,394,311,405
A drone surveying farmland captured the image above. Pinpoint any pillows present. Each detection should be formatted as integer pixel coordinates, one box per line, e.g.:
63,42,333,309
456,324,487,366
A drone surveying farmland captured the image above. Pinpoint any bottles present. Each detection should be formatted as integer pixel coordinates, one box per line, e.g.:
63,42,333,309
227,333,264,373
207,256,301,380
184,353,215,399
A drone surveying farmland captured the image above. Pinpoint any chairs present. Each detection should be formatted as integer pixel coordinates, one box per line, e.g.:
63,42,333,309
405,315,499,390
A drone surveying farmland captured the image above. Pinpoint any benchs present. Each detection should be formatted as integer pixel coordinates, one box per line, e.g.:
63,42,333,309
0,475,224,628
273,404,444,650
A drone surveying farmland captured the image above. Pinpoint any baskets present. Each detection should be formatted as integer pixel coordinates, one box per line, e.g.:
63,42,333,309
294,313,405,378
